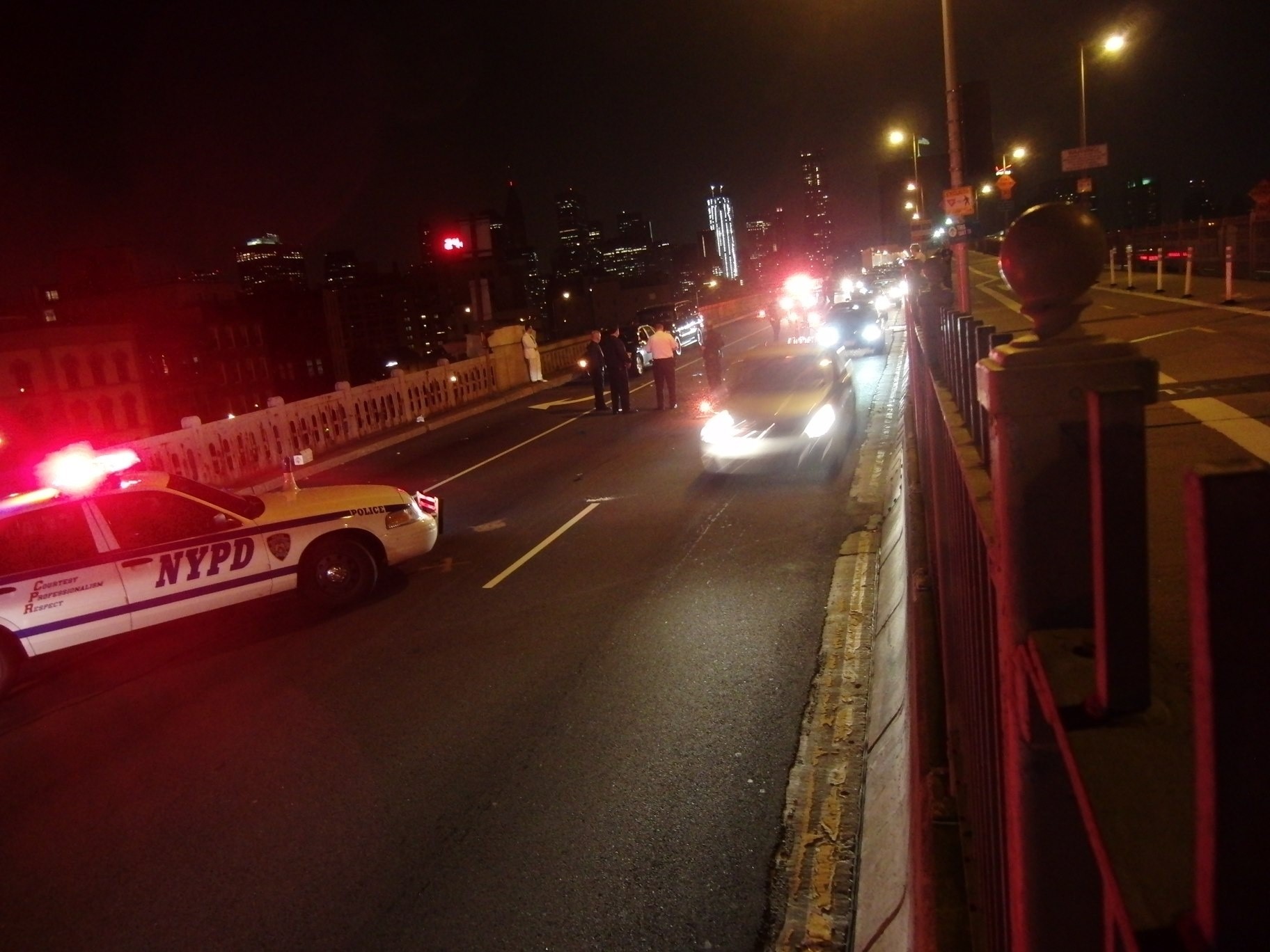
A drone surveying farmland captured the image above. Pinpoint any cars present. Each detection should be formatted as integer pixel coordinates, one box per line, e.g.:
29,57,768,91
700,345,859,477
1,449,440,700
772,262,905,356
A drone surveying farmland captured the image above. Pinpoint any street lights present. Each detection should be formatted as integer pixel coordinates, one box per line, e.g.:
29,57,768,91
1078,28,1125,210
975,183,994,236
996,146,1025,235
889,130,929,220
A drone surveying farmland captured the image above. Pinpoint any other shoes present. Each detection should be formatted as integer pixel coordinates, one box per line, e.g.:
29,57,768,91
538,377,548,382
605,406,613,411
659,406,665,412
614,409,640,414
671,404,678,409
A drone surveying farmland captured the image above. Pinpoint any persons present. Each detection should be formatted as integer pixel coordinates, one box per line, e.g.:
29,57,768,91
701,321,725,390
822,275,836,304
605,323,639,413
588,331,612,410
941,239,953,291
645,324,679,410
766,295,781,341
522,325,547,384
909,243,925,275
